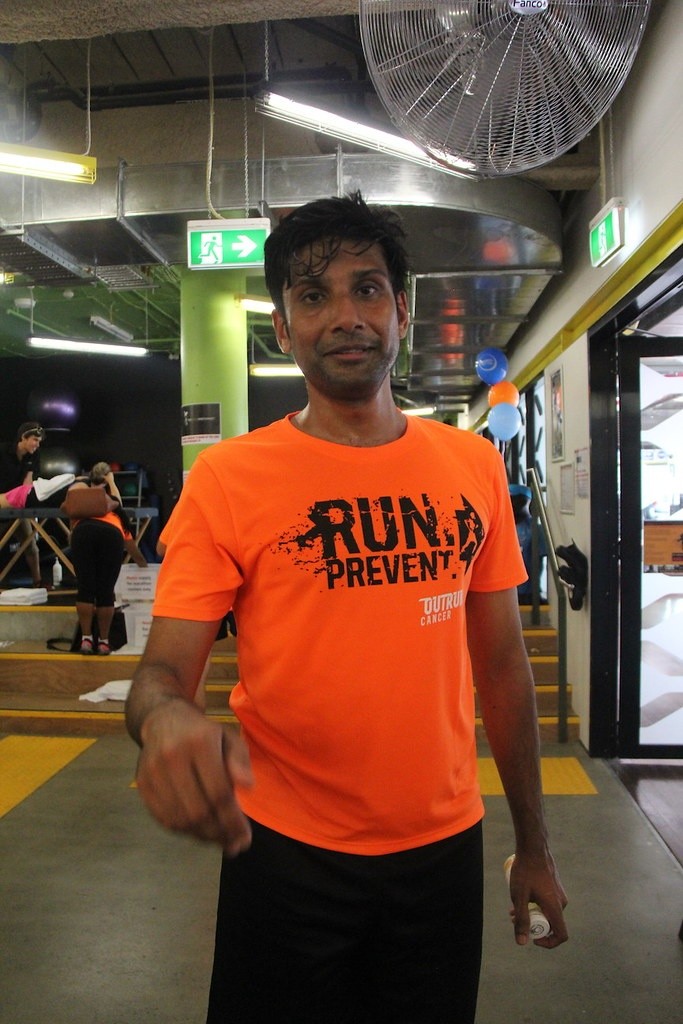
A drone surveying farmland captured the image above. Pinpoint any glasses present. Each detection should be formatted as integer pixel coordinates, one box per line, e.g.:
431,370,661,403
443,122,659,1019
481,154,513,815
22,426,43,437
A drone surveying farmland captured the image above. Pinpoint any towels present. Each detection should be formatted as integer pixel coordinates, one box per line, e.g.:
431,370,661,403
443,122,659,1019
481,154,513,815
32,473,75,501
78,679,133,704
0,587,47,606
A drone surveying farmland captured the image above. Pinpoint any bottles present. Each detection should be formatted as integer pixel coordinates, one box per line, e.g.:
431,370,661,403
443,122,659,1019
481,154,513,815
53,556,64,589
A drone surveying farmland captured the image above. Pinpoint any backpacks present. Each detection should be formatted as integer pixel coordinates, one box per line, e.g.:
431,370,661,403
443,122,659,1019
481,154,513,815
59,472,120,518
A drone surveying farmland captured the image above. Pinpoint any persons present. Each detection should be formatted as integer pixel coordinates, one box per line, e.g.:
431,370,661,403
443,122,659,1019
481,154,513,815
443,418,453,425
0,422,148,654
510,484,548,605
122,190,567,1024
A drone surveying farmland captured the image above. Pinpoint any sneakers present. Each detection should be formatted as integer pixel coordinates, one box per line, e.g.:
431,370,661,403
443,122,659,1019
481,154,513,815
79,638,95,655
95,641,111,655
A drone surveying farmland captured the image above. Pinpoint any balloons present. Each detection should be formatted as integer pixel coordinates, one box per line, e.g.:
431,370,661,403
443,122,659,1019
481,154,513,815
475,347,522,440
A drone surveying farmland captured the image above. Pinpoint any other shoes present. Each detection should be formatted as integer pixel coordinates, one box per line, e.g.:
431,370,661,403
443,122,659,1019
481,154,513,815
33,580,55,591
